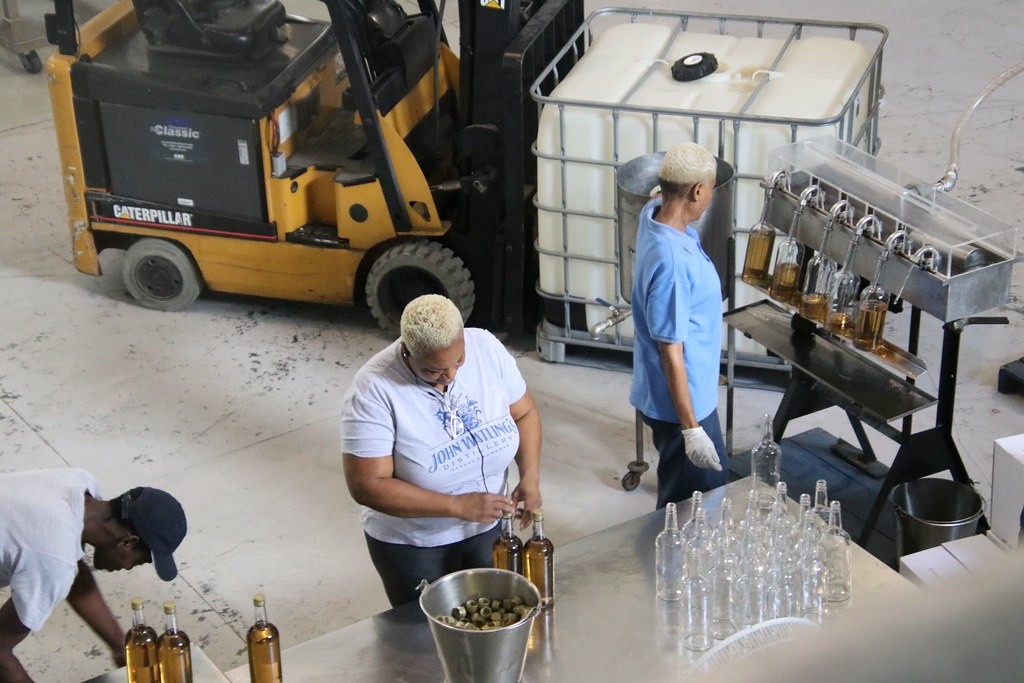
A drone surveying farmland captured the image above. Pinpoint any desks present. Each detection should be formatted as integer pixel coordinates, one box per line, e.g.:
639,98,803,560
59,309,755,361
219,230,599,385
218,472,1024,683
79,641,233,683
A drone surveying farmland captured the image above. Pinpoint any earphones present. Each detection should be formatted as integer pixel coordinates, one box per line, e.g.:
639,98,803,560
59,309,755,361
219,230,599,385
402,346,410,359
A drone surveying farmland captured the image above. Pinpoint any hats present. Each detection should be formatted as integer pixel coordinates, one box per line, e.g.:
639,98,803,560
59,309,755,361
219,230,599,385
123,484,185,580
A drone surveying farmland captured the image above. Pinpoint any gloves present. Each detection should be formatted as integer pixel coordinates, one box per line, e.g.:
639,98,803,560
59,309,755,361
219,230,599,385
683,425,725,473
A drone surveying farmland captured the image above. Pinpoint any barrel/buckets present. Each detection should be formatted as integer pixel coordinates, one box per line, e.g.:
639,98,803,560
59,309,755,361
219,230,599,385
888,476,987,568
414,568,557,683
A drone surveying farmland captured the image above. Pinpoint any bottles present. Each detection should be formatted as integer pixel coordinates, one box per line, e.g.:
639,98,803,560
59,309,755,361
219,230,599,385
521,507,555,611
852,256,892,352
122,598,159,683
157,601,194,683
742,194,776,285
799,225,838,322
751,414,783,510
768,210,806,302
821,240,861,335
656,479,852,652
247,594,282,682
492,512,525,577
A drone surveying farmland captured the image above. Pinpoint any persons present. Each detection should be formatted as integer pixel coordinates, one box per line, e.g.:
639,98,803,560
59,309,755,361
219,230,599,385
0,468,186,683
629,142,730,511
341,293,542,609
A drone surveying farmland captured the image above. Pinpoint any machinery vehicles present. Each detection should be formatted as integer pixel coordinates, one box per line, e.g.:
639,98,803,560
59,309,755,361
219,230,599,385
44,1,585,350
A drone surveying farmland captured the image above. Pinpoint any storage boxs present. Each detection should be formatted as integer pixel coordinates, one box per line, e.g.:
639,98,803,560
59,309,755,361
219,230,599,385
990,433,1024,549
899,545,968,588
940,534,1004,573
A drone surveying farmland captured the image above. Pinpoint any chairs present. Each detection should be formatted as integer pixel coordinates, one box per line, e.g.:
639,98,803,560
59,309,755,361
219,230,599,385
130,0,287,63
